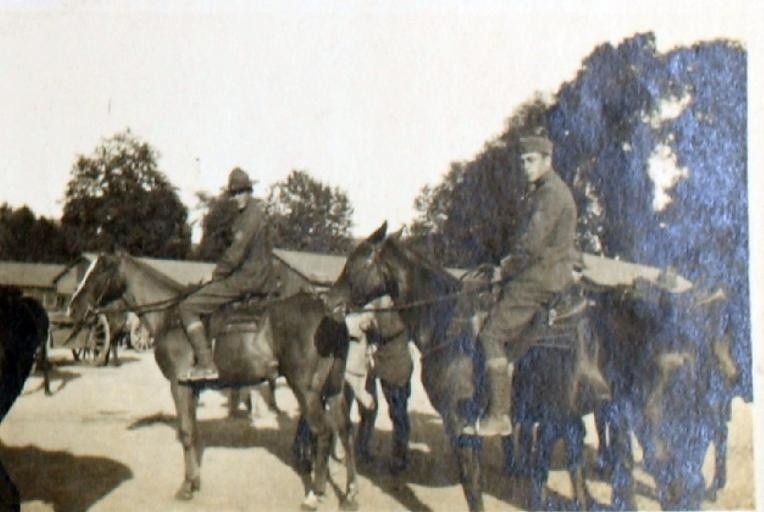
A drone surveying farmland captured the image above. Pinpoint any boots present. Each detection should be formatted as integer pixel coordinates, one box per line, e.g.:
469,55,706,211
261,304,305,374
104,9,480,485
176,324,220,384
462,358,513,437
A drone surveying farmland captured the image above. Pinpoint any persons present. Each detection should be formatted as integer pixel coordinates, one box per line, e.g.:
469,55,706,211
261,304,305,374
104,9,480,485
462,133,577,437
177,168,273,383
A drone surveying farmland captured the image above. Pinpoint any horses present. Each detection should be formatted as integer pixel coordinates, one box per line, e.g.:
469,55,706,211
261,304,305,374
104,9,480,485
66,234,351,511
325,220,743,512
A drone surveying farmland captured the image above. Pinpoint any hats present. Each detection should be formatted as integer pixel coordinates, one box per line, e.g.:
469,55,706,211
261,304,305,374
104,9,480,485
221,168,257,190
517,135,554,155
